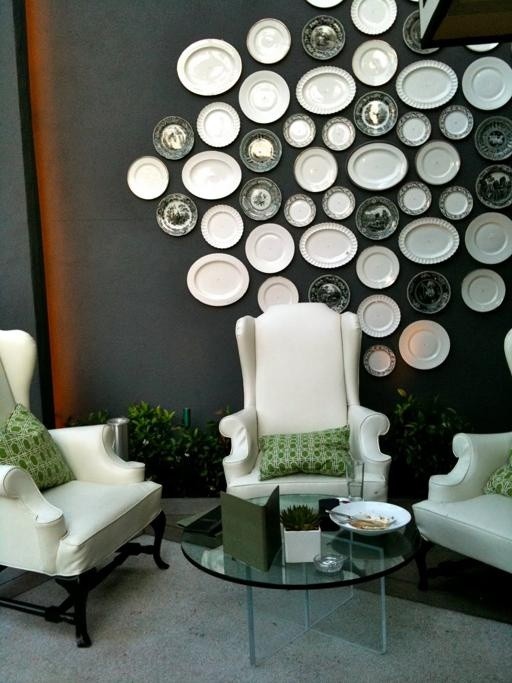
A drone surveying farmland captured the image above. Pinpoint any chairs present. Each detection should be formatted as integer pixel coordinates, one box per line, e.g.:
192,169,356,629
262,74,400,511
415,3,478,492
0,330,171,648
413,329,512,592
219,302,392,504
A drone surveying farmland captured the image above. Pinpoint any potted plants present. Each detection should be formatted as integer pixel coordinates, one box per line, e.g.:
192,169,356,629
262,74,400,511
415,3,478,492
279,504,322,564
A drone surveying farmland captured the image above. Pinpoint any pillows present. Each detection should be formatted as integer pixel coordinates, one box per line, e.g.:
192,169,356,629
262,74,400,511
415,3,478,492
482,447,512,498
258,425,355,481
0,402,77,490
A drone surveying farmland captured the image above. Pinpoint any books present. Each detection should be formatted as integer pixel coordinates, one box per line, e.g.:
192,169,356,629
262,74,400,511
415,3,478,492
182,506,221,536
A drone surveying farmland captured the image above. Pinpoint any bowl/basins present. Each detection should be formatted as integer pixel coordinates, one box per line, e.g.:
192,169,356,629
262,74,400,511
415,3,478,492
313,553,344,572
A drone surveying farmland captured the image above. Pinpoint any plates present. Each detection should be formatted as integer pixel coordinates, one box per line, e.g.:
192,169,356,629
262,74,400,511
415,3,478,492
240,128,283,171
464,212,512,264
236,174,283,221
461,269,506,312
322,186,355,220
152,117,194,160
293,147,339,193
283,113,317,148
351,0,397,35
403,9,440,54
466,43,500,51
415,140,461,186
352,39,398,87
296,65,356,115
355,196,400,240
397,111,432,146
245,223,295,274
330,501,412,536
439,105,474,140
300,15,346,61
238,70,291,124
354,91,398,137
321,116,356,151
363,345,396,377
356,245,400,289
309,275,350,313
357,294,402,338
197,102,240,147
186,253,251,306
127,156,170,201
157,193,198,237
246,18,291,64
176,39,243,95
308,0,343,8
345,140,408,190
282,194,317,226
398,217,460,265
398,182,431,214
398,320,450,370
395,60,458,110
299,222,358,268
406,271,452,313
462,56,512,110
475,116,512,160
201,205,244,250
439,186,474,221
257,276,299,313
181,151,241,200
475,165,512,209
